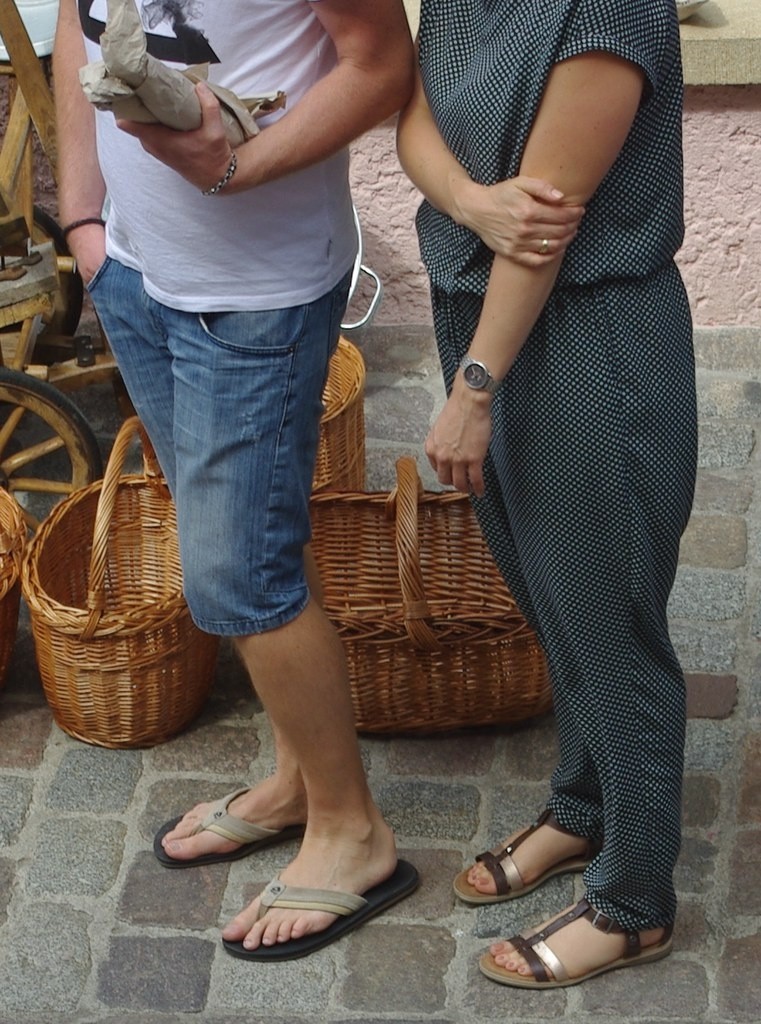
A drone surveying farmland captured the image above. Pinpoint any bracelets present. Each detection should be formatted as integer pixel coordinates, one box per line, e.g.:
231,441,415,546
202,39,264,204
204,153,236,197
59,218,107,240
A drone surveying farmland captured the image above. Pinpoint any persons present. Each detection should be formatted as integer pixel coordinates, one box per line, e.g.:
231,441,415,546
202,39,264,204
399,1,699,990
54,0,420,965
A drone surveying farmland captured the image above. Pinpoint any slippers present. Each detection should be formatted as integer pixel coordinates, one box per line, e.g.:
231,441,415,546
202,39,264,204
154,786,306,868
220,859,420,963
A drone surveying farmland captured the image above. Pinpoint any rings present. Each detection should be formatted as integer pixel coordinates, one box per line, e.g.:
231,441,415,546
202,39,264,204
540,239,548,256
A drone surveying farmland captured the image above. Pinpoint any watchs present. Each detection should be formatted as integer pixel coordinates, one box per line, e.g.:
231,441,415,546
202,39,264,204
460,355,502,395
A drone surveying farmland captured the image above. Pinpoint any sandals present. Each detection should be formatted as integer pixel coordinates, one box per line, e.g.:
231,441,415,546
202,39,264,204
480,897,676,987
451,808,598,903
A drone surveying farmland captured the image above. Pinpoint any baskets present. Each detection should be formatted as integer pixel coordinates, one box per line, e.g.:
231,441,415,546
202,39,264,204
304,456,554,734
22,416,217,750
311,334,367,494
0,486,28,683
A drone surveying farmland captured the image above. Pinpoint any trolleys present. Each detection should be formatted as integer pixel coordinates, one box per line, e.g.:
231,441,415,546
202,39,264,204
0,1,134,539
